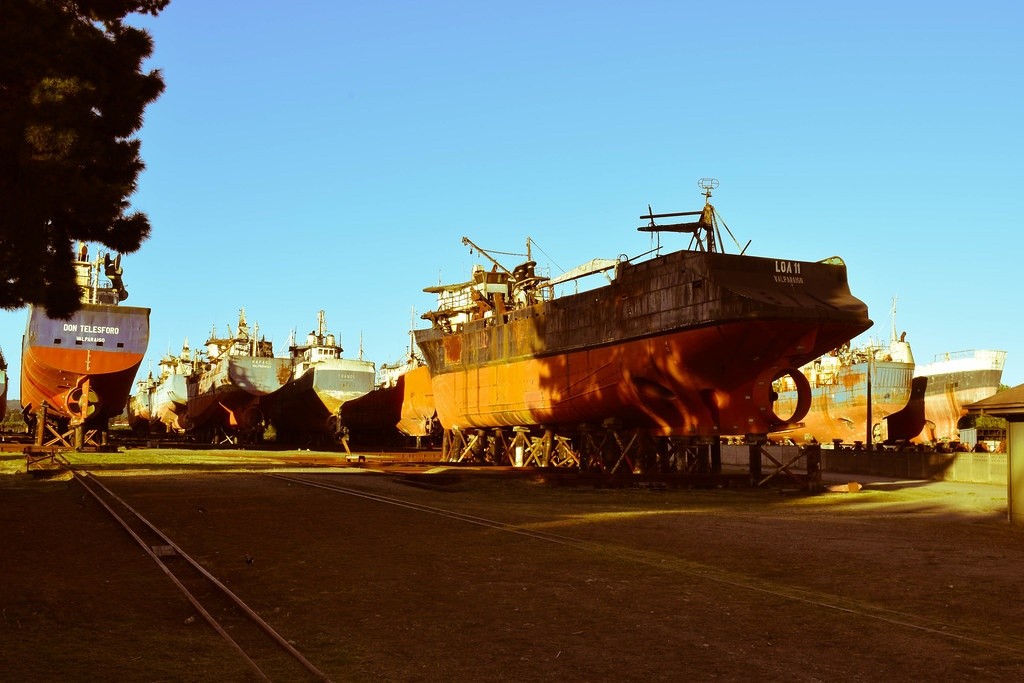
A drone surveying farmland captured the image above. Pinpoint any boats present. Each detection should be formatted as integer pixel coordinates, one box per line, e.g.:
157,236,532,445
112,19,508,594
21,241,151,435
413,176,874,436
261,307,1007,445
128,305,293,437
